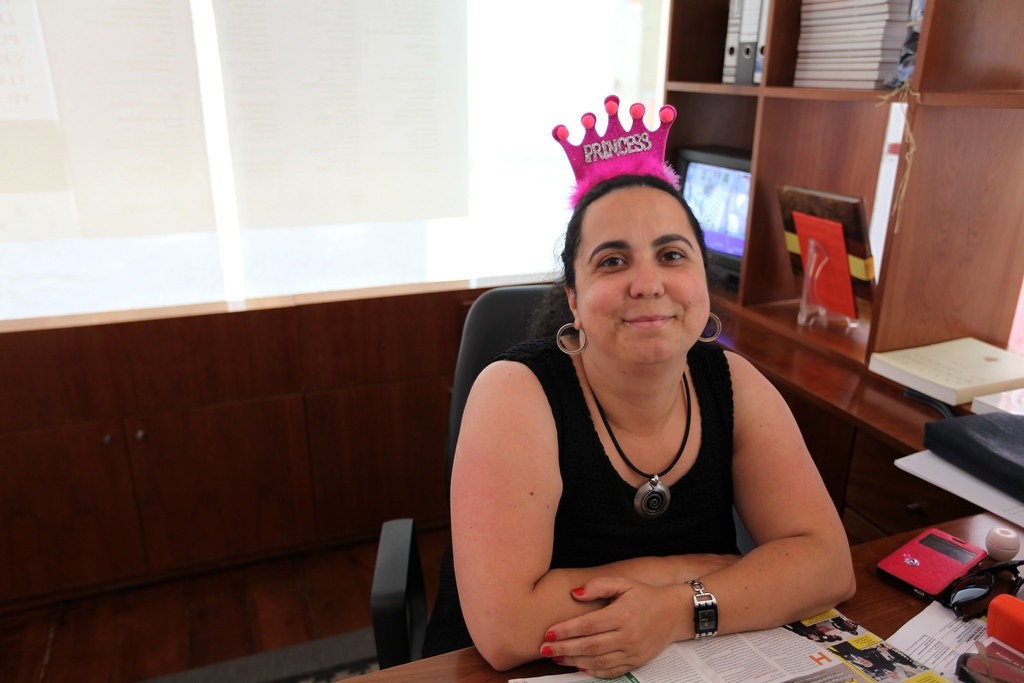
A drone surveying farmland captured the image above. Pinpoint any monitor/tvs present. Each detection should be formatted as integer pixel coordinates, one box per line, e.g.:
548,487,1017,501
670,147,753,292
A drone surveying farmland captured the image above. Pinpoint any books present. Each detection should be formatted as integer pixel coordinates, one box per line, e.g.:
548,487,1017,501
893,449,1024,530
870,335,1024,406
970,387,1024,418
507,607,949,683
711,0,928,91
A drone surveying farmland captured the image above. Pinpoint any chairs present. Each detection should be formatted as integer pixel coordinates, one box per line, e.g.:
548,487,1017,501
367,284,764,672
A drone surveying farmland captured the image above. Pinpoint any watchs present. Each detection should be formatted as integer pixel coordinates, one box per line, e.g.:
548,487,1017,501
684,578,719,640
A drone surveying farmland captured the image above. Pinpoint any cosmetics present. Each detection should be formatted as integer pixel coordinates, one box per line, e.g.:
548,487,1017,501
984,526,1020,562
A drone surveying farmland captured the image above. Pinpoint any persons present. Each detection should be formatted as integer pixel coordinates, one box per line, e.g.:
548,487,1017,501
448,97,857,680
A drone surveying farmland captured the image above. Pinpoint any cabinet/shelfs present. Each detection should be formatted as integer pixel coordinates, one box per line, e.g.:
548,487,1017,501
660,0,1024,416
839,428,988,548
774,380,856,520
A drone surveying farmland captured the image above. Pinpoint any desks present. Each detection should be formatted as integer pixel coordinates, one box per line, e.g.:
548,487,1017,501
327,510,1024,683
